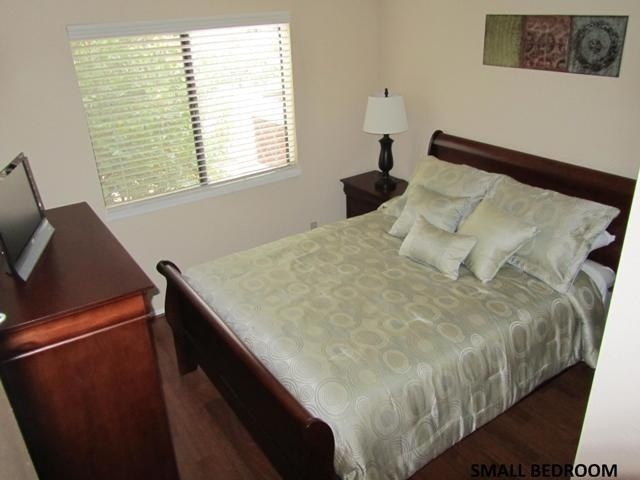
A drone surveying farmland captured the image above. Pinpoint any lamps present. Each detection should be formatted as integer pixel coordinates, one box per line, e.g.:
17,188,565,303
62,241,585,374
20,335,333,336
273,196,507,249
362,88,409,190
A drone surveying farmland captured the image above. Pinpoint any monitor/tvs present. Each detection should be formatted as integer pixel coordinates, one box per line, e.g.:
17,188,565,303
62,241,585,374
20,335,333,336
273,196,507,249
0,150,55,284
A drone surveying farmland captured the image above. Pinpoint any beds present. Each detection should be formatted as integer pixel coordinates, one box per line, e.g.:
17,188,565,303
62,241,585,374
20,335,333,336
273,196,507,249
157,130,636,480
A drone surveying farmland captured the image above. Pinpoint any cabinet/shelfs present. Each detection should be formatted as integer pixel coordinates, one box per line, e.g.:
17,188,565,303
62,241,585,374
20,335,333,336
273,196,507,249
0,202,181,480
339,170,408,219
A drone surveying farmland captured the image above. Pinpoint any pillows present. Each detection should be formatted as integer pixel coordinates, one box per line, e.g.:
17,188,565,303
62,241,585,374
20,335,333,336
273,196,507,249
398,215,475,281
403,155,500,229
457,200,539,283
471,175,620,293
389,183,471,239
